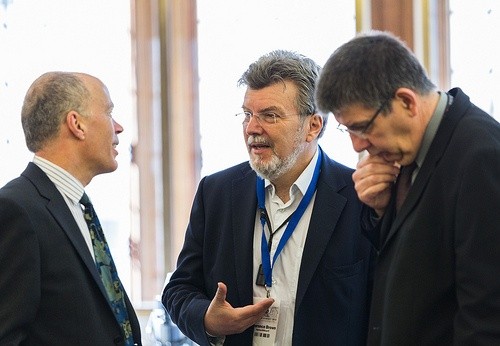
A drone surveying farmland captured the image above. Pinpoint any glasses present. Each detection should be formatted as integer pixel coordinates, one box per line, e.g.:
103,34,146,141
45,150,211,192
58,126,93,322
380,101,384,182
236,111,311,126
336,93,397,139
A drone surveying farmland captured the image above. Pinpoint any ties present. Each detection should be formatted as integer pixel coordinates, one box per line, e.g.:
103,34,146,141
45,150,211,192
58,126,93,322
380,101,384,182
77,192,134,346
394,159,418,215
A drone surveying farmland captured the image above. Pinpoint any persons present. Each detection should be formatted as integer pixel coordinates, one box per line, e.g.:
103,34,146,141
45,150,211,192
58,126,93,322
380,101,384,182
162,51,373,345
316,34,499,346
1,70,143,346
